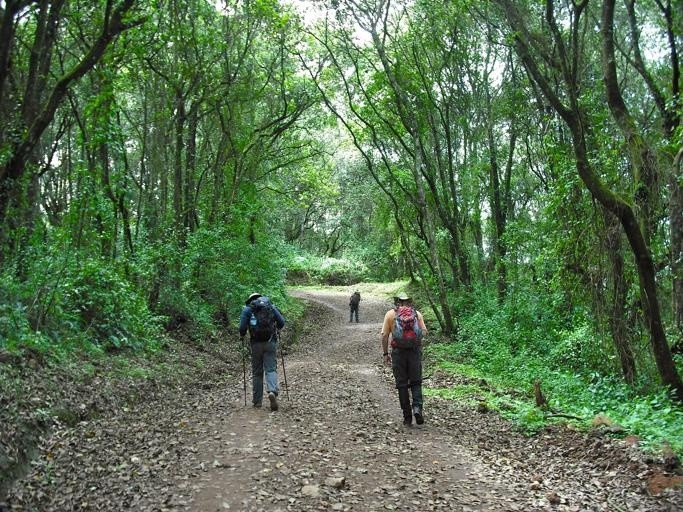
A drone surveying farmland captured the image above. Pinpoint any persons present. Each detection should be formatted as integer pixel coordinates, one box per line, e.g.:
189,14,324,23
347,290,360,323
379,292,427,426
237,292,284,411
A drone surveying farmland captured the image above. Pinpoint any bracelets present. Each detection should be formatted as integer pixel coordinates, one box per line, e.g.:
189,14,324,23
382,352,388,356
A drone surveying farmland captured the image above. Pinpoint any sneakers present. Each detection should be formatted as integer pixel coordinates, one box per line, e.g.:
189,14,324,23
254,400,262,407
268,390,279,411
414,404,425,425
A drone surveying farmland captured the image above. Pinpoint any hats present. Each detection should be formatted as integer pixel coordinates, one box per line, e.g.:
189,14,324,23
393,291,414,304
244,292,261,305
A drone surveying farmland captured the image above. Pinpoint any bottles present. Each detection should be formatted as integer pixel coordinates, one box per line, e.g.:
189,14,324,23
249,313,257,326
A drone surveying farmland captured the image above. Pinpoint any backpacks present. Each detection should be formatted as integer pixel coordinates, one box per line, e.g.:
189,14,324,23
248,295,277,343
392,305,422,350
